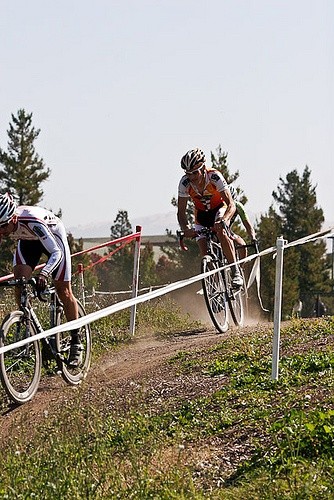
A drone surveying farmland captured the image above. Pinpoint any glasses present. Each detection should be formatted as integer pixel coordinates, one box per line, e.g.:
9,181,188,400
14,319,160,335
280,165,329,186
1,223,9,229
184,163,204,178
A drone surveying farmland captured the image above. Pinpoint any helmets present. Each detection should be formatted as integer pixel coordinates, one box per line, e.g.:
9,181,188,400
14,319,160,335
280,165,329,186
228,185,236,198
181,148,206,171
0,192,17,227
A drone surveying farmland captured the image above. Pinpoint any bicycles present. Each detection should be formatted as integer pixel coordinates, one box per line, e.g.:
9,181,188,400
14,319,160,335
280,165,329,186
0,260,93,405
176,217,260,334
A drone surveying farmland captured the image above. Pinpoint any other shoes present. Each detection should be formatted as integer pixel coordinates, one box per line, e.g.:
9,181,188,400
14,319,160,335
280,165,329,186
231,272,243,286
212,295,222,312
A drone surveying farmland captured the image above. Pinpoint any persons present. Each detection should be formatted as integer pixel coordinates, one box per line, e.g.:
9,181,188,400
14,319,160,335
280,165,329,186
177,149,257,312
0,192,83,368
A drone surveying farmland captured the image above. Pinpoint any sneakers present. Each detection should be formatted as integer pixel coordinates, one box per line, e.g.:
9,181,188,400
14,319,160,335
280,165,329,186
67,339,84,367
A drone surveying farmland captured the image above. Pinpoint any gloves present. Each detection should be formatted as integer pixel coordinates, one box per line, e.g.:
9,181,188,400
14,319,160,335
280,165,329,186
34,274,48,293
